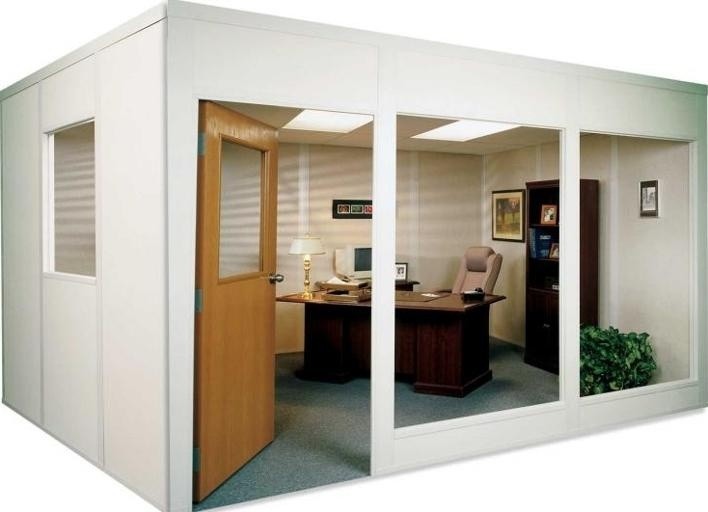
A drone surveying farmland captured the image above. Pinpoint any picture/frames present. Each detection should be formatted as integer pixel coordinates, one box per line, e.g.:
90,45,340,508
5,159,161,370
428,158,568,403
395,262,407,281
492,189,525,243
333,199,372,219
540,205,557,224
640,180,658,217
549,243,559,259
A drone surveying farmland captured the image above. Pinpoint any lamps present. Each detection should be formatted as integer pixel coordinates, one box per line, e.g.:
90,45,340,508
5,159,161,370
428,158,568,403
288,232,325,300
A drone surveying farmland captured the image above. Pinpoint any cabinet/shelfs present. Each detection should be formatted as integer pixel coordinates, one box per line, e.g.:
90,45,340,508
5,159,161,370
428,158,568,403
395,280,420,291
524,179,599,374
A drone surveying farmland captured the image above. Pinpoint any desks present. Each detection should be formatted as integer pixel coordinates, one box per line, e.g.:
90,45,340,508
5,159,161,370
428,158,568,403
276,290,506,398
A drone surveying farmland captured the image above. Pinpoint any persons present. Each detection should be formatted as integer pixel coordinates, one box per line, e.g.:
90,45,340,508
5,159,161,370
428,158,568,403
397,268,404,278
544,209,553,220
552,246,559,257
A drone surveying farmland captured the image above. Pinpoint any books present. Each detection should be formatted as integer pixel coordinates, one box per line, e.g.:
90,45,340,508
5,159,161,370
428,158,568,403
528,228,551,258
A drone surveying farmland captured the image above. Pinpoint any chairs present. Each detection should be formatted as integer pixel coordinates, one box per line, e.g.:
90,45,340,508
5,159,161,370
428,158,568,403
452,246,502,294
